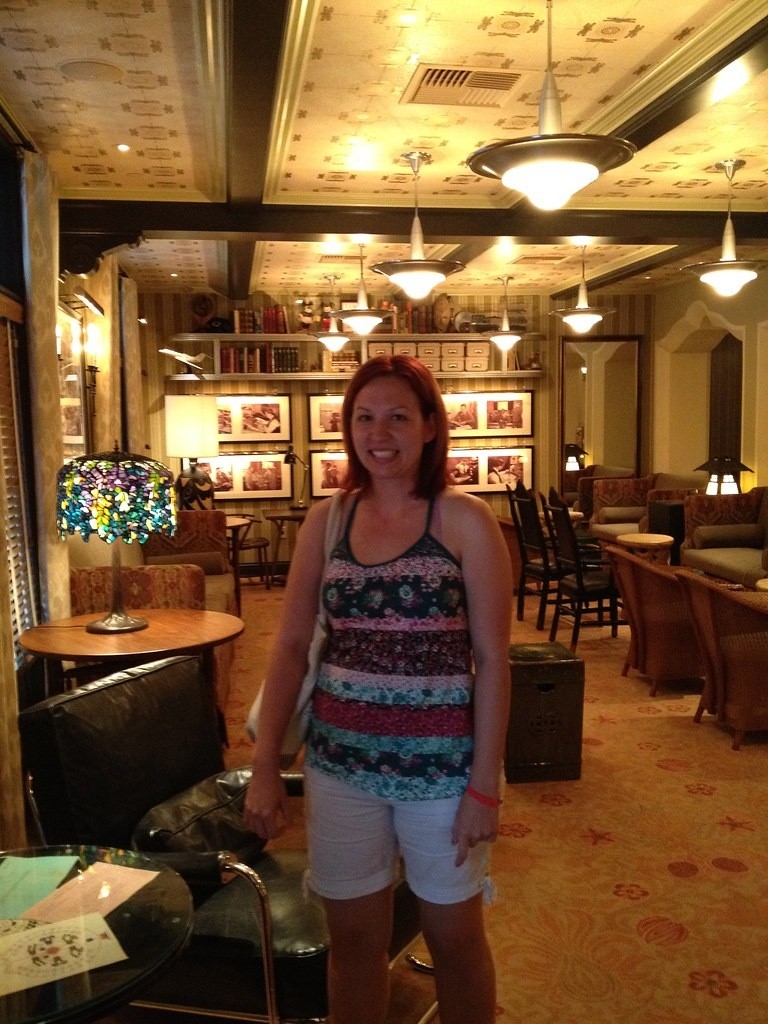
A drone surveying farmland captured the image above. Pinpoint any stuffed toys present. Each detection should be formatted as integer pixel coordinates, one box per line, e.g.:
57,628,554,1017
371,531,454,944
299,301,335,334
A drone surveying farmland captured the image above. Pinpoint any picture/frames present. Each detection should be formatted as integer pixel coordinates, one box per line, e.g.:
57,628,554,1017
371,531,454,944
181,390,538,503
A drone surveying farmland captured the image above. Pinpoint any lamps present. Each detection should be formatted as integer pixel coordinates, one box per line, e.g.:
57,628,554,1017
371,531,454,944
53,285,219,633
581,364,587,381
316,1,768,353
693,457,755,496
564,444,589,471
283,445,310,510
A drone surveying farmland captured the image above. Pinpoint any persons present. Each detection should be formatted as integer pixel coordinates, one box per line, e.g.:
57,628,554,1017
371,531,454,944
243,355,512,1024
217,404,285,435
329,413,339,431
202,461,281,491
320,461,340,488
444,403,523,484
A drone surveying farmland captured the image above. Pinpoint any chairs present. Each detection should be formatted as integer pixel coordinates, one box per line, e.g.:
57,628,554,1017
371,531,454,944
225,512,271,592
18,652,441,1024
505,480,768,751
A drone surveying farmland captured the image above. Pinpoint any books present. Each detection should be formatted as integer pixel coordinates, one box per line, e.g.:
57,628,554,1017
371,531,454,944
218,302,299,373
332,350,359,372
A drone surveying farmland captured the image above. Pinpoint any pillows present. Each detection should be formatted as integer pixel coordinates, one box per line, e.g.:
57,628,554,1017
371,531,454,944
131,765,267,908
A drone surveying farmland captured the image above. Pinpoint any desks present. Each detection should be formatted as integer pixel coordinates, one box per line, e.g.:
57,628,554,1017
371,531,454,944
226,516,251,608
0,844,195,1024
537,510,584,525
617,532,674,565
17,608,247,699
263,510,306,585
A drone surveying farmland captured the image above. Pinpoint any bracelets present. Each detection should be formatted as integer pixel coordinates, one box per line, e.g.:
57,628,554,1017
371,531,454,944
466,785,504,808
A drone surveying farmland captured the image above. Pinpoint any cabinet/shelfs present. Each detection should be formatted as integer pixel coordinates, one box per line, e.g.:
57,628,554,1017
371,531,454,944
165,331,551,382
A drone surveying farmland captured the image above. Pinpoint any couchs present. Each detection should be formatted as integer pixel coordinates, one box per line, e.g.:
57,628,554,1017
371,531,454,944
563,462,636,520
680,486,768,595
70,509,241,727
588,473,714,564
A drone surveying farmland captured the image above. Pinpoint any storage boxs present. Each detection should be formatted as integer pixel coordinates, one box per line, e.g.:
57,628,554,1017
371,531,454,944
368,343,490,372
